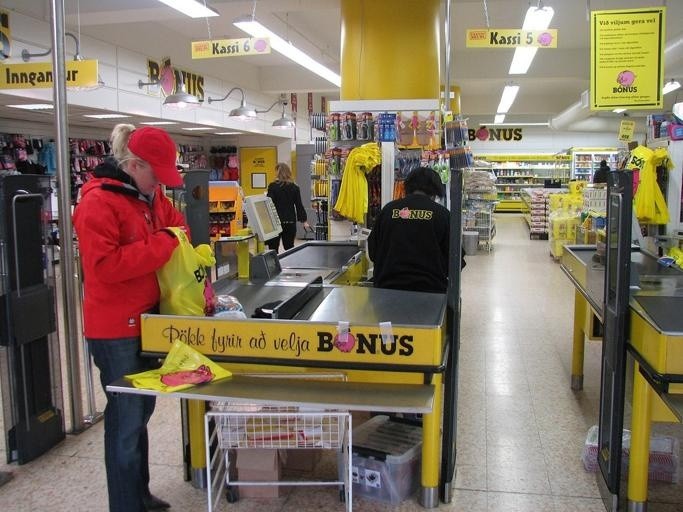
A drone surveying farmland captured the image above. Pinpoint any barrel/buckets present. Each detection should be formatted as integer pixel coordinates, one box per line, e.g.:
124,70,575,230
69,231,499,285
462,230,479,256
329,216,353,242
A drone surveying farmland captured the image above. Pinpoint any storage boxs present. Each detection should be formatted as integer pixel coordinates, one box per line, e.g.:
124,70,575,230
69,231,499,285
235,449,287,498
337,415,423,505
278,448,323,472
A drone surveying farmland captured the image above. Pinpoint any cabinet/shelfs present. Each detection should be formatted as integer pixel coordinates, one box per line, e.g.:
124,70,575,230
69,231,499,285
573,153,618,184
488,158,569,203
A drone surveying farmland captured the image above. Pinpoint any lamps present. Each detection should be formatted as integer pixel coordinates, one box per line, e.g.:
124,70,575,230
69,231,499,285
253,101,292,128
232,1,342,88
138,67,198,109
207,87,256,120
21,32,103,94
660,79,681,95
506,1,553,75
158,0,222,19
496,80,520,114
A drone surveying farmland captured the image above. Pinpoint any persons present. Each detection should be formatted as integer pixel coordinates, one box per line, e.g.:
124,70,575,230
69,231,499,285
264,162,310,254
367,166,466,293
71,123,192,512
593,160,613,187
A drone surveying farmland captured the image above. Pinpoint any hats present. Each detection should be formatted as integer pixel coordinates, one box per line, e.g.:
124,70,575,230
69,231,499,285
127,126,183,188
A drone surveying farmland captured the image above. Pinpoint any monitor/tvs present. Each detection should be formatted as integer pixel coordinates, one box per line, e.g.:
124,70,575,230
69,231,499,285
247,195,284,255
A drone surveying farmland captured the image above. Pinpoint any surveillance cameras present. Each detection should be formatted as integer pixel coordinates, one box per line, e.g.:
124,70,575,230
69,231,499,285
281,96,288,106
